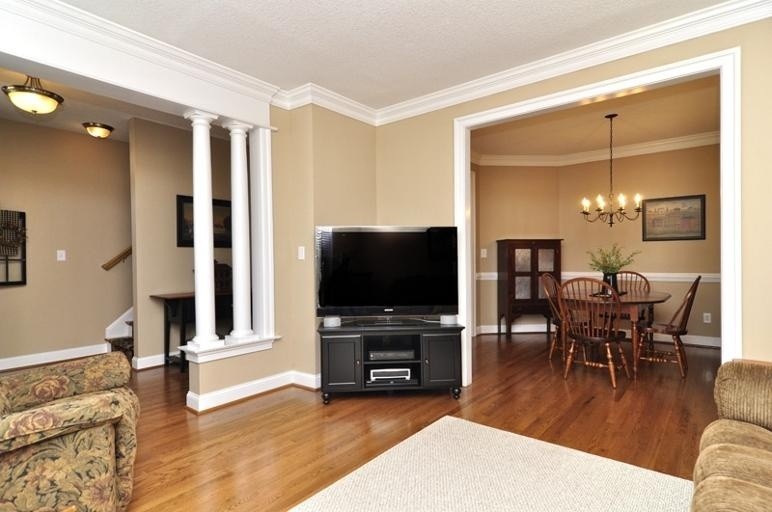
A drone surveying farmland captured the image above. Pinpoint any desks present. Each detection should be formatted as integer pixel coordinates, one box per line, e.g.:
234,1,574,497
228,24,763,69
149,290,232,374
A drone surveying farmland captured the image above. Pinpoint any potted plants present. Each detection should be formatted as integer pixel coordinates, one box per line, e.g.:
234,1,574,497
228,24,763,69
584,239,643,294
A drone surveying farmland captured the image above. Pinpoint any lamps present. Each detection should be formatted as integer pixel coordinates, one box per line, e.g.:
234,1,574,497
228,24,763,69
1,72,65,118
80,117,118,140
577,112,643,228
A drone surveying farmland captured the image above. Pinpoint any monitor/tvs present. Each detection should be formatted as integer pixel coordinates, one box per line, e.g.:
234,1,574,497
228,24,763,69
313,224,459,325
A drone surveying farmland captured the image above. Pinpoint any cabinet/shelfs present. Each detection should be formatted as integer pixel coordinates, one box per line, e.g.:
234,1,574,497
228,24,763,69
494,238,565,340
317,321,466,406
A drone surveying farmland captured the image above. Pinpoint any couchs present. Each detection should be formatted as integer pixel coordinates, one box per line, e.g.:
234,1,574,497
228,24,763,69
689,352,772,511
1,349,143,511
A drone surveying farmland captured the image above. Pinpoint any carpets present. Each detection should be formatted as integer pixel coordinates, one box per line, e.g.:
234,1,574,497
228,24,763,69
281,411,697,511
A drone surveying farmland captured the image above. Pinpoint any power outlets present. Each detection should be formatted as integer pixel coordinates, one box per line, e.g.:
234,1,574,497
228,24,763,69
704,313,711,324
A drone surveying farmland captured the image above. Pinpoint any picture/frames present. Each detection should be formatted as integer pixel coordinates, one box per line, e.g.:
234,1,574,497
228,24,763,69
639,194,707,242
175,194,232,249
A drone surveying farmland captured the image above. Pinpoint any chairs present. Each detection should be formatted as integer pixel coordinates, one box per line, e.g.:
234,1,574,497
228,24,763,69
540,273,595,368
556,274,631,390
598,269,653,356
630,275,704,381
